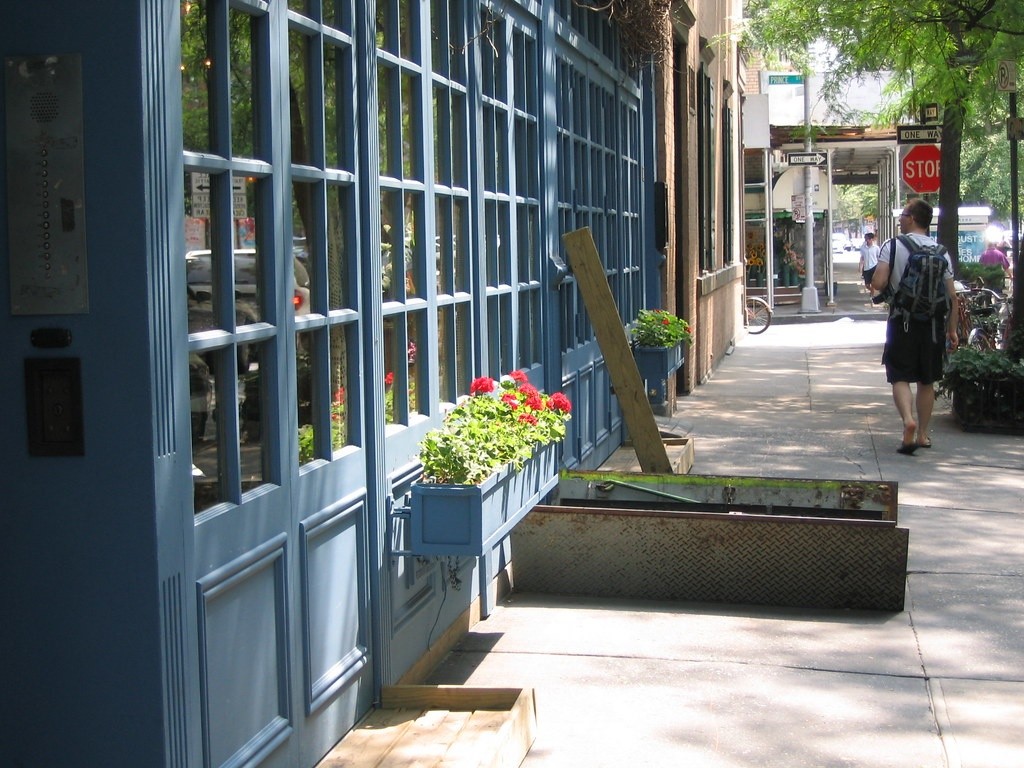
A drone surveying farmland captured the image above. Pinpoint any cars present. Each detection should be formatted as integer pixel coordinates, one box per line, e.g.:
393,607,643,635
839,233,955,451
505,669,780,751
832,232,863,255
185,235,460,448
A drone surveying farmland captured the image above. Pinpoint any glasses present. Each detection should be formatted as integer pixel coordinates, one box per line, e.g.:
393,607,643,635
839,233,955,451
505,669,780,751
898,213,910,219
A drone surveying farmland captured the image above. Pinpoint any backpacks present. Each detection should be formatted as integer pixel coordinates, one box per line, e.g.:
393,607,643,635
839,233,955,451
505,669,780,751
889,234,953,323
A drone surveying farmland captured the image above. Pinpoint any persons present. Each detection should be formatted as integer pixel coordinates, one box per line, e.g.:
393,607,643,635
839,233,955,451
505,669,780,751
979,238,1013,309
871,198,959,454
859,233,880,301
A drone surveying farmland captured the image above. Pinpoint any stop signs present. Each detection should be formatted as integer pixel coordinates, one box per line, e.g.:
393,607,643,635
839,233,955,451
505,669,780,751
901,144,942,194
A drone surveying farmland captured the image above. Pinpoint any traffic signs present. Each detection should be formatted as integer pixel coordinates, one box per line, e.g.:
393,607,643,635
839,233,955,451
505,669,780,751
895,124,944,143
787,151,828,165
920,102,939,122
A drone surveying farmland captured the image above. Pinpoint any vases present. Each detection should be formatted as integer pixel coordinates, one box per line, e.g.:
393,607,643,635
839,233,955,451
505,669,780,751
411,434,558,556
631,344,684,379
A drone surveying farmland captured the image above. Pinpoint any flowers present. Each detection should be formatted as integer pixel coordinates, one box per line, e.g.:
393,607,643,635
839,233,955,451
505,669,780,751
625,308,692,347
414,371,571,485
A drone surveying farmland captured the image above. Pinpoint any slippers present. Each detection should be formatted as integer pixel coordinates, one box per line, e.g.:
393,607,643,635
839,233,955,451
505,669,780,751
916,436,932,448
896,440,919,454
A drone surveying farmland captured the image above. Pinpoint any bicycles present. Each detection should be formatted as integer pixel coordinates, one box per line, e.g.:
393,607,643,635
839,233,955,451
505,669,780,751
950,276,1015,355
746,294,773,334
